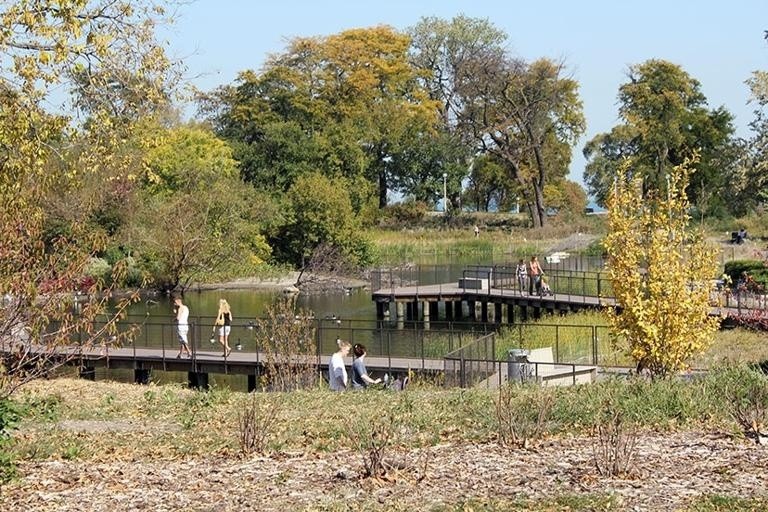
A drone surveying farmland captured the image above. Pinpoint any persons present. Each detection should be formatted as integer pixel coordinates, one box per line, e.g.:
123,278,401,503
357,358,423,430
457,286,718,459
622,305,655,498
328,341,352,390
540,278,551,292
351,344,382,388
212,299,233,357
515,260,527,297
740,229,747,238
173,297,192,359
529,256,544,296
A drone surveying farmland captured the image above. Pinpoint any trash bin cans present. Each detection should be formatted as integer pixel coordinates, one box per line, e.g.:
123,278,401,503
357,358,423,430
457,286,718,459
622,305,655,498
508,349,529,385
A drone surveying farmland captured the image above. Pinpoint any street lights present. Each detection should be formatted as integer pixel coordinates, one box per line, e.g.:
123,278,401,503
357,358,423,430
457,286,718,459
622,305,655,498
442,172,448,211
516,197,521,214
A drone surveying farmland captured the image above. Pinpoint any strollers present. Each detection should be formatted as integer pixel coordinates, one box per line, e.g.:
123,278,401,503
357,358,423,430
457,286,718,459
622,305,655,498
534,274,553,296
